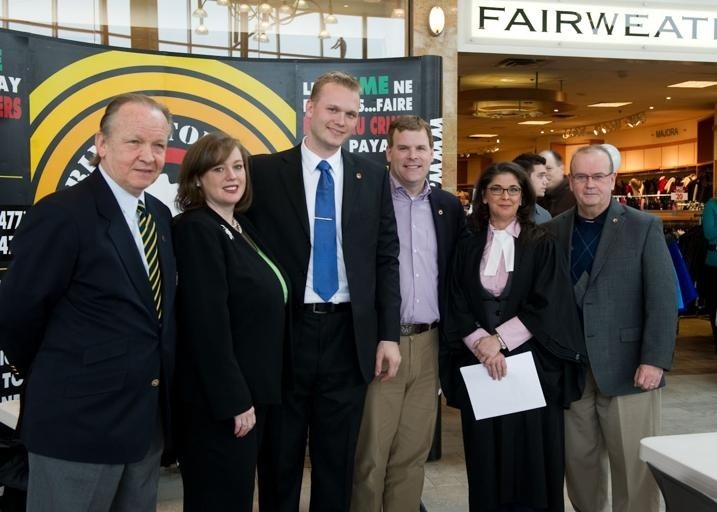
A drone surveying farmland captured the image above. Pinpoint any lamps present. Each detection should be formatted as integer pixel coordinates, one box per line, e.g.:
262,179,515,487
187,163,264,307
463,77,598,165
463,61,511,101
561,111,645,139
193,0,338,39
428,6,444,38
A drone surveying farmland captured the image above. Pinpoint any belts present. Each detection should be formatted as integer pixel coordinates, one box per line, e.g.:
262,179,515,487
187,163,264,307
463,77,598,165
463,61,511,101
399,320,440,336
305,302,351,315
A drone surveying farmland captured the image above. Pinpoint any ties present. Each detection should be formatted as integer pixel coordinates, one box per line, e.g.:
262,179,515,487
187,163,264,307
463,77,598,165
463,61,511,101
314,161,339,302
137,199,163,330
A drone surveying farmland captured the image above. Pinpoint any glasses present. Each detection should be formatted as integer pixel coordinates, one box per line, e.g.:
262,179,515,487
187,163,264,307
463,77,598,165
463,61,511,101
570,173,610,183
490,185,520,196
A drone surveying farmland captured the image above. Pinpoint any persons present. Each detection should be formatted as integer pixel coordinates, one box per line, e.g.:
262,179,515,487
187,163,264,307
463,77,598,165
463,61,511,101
167,129,291,511
346,117,465,511
232,71,402,512
702,197,717,347
434,160,569,512
0,93,179,512
538,149,576,214
511,151,553,226
529,145,678,512
626,177,645,210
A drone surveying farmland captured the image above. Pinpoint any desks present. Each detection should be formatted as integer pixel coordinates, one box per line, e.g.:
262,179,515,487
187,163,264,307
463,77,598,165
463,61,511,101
640,431,717,512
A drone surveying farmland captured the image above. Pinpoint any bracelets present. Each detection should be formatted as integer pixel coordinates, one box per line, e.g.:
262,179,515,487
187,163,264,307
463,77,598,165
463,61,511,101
496,333,507,351
